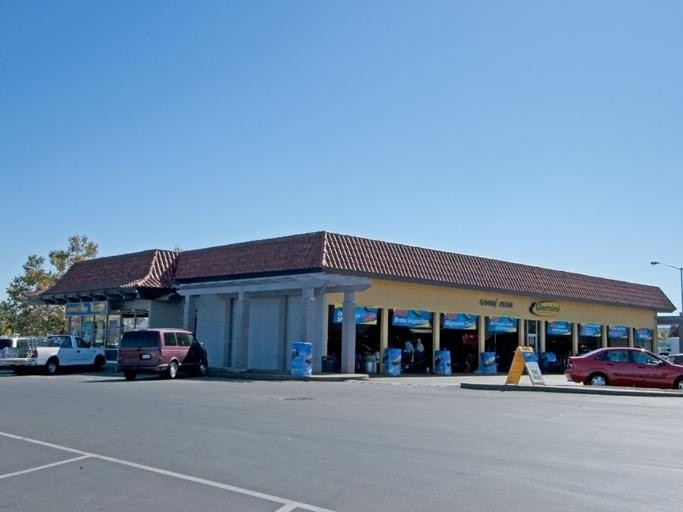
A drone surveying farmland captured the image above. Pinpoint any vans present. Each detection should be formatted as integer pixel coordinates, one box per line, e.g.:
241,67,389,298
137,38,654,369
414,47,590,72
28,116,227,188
117,326,211,379
0,335,47,351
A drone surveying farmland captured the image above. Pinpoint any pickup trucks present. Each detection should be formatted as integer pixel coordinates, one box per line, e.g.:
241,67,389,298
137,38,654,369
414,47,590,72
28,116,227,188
0,334,108,375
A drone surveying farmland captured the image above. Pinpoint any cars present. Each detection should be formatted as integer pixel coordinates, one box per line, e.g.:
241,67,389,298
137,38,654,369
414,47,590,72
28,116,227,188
662,353,683,366
564,346,683,389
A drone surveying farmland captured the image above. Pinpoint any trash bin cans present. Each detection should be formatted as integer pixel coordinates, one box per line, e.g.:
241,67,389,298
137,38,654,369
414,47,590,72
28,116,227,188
361,356,376,374
547,361,565,374
321,356,337,373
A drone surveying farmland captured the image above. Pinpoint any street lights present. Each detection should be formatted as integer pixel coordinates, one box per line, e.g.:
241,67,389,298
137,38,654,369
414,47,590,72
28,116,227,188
649,261,683,353
